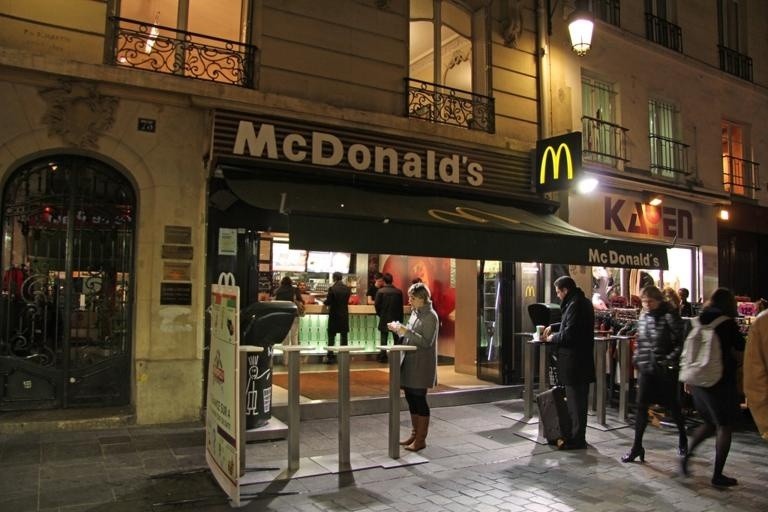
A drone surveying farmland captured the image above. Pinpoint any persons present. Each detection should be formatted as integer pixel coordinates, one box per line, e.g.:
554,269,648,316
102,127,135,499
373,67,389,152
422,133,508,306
322,272,351,362
375,273,404,361
275,277,304,366
387,282,439,452
366,273,384,305
679,289,691,317
542,276,594,449
680,288,746,486
622,285,687,462
743,309,768,444
294,281,315,305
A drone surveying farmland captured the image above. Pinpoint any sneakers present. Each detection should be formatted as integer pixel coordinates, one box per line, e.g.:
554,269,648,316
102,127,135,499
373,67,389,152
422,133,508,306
559,439,588,449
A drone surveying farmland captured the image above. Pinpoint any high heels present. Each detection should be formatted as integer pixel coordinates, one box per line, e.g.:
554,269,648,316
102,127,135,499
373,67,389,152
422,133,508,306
678,438,689,458
621,447,645,462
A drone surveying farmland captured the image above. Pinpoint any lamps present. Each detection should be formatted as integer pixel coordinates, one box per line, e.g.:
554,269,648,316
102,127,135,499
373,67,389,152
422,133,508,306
143,0,160,55
642,190,663,207
712,205,730,221
549,0,595,57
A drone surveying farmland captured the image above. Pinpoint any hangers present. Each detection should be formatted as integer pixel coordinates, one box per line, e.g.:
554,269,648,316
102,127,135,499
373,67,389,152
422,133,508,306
737,317,751,333
595,315,639,326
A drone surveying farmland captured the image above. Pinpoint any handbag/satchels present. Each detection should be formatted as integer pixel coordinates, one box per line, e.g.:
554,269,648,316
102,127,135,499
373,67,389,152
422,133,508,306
293,300,306,317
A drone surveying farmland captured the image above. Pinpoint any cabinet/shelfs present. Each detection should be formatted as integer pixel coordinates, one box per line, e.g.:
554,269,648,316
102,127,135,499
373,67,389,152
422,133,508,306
483,273,499,327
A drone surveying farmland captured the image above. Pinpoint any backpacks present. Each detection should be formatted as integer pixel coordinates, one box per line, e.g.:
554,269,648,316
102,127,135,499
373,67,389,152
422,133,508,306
678,315,732,388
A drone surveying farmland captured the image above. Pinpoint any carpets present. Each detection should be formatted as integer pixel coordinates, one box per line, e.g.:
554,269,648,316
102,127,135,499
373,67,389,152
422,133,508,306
272,370,458,399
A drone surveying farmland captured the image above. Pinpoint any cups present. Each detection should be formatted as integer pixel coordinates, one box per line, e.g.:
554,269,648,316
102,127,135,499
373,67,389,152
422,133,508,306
536,326,545,340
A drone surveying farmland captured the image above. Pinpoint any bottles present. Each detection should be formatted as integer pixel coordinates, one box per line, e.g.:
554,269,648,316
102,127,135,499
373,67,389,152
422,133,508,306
326,341,328,346
317,316,320,327
350,341,354,346
300,318,303,326
308,316,312,327
373,340,376,345
350,314,355,328
350,328,354,338
358,341,360,344
364,328,368,338
316,283,328,290
308,328,312,339
300,328,303,339
307,341,311,345
317,342,320,344
356,328,361,340
325,316,329,327
364,315,368,327
372,328,376,339
356,314,360,327
389,336,391,344
316,328,320,340
373,315,377,327
364,341,367,344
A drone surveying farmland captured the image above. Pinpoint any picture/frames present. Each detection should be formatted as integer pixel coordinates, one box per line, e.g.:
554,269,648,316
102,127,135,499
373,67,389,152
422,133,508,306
258,237,273,262
258,263,273,272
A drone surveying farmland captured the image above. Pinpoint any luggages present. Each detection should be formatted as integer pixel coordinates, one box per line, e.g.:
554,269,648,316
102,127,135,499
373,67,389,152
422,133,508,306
536,366,572,445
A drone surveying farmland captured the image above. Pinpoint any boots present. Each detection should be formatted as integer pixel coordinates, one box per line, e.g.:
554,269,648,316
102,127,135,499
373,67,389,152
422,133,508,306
399,414,418,445
711,450,737,486
404,416,429,451
